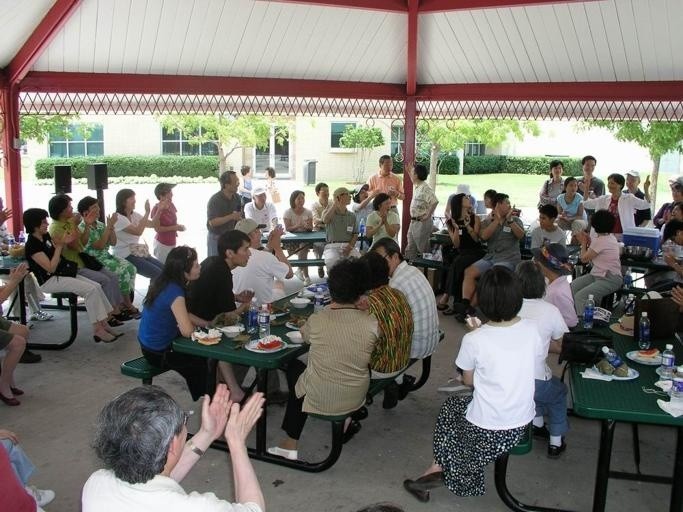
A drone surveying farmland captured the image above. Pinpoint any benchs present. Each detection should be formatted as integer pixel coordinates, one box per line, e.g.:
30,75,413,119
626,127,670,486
42,290,134,350
412,258,446,297
285,259,326,266
601,287,650,309
494,421,532,511
0,255,42,349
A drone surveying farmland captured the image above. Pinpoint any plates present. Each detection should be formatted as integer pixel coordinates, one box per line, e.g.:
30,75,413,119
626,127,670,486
244,337,286,354
587,349,664,380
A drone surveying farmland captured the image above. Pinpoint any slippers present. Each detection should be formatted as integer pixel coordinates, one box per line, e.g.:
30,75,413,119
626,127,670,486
132,308,142,320
437,303,449,311
441,308,455,316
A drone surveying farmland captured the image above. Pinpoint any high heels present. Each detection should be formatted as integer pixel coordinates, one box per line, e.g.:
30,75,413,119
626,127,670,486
110,330,125,338
93,331,118,343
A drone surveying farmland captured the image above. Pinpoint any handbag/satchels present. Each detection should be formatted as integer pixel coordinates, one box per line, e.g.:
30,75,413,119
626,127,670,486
634,292,679,340
78,252,105,272
128,243,150,259
557,330,614,368
52,258,79,278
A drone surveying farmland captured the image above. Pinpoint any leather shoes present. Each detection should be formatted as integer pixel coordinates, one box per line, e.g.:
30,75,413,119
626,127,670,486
10,384,24,396
398,374,416,401
0,391,21,406
382,379,399,410
403,479,430,504
352,406,368,421
415,470,445,490
343,418,362,445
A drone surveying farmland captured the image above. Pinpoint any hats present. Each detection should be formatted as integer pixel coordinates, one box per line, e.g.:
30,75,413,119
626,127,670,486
530,243,575,275
455,183,470,195
234,219,267,236
352,184,369,204
624,168,640,177
252,186,265,196
668,176,683,186
160,183,175,192
333,187,356,197
609,312,635,336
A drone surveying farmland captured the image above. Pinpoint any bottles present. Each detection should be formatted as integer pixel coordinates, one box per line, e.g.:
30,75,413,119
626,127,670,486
7,231,26,248
244,286,325,339
358,217,366,238
524,228,531,250
581,293,683,415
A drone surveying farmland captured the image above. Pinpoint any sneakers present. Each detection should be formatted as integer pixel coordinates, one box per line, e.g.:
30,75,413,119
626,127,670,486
454,298,470,314
318,267,325,278
547,436,567,460
531,422,550,442
18,320,33,328
29,311,55,321
18,349,42,364
304,277,313,287
436,378,474,396
107,317,125,327
294,270,305,281
268,447,298,460
25,483,56,508
267,391,289,404
112,311,134,322
454,305,476,324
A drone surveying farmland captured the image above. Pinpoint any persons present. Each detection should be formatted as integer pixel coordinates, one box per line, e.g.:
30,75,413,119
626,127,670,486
403,265,542,503
80,383,268,512
136,155,440,459
1,184,186,407
435,154,681,458
0,429,56,512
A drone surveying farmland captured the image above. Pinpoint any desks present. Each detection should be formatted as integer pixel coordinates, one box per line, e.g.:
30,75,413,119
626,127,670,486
173,281,333,464
620,254,681,270
429,235,581,260
565,316,683,512
261,230,370,243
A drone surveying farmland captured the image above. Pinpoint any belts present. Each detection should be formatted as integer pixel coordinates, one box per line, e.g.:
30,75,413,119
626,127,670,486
411,217,423,221
326,240,351,244
391,206,396,208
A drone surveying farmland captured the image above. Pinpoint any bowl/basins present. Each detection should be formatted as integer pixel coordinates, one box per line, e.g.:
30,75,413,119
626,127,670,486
220,325,245,339
288,296,311,310
622,245,653,260
284,330,303,345
